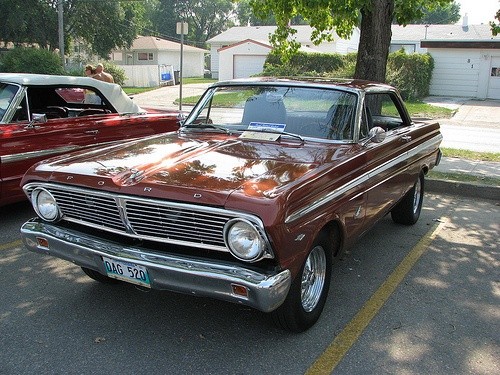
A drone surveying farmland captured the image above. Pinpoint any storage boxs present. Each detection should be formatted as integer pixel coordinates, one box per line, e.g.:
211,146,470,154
162,73,170,80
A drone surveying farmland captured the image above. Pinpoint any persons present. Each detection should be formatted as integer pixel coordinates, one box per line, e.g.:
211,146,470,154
83,63,114,94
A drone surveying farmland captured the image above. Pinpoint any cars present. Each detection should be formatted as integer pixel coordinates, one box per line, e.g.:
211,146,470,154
20,76,447,335
0,73,214,207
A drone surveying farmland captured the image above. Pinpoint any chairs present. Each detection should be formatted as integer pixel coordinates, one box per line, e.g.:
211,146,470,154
325,104,373,138
242,94,286,124
78,108,111,116
41,106,69,119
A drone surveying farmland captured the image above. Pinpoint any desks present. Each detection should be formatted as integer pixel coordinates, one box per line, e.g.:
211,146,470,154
160,79,173,86
